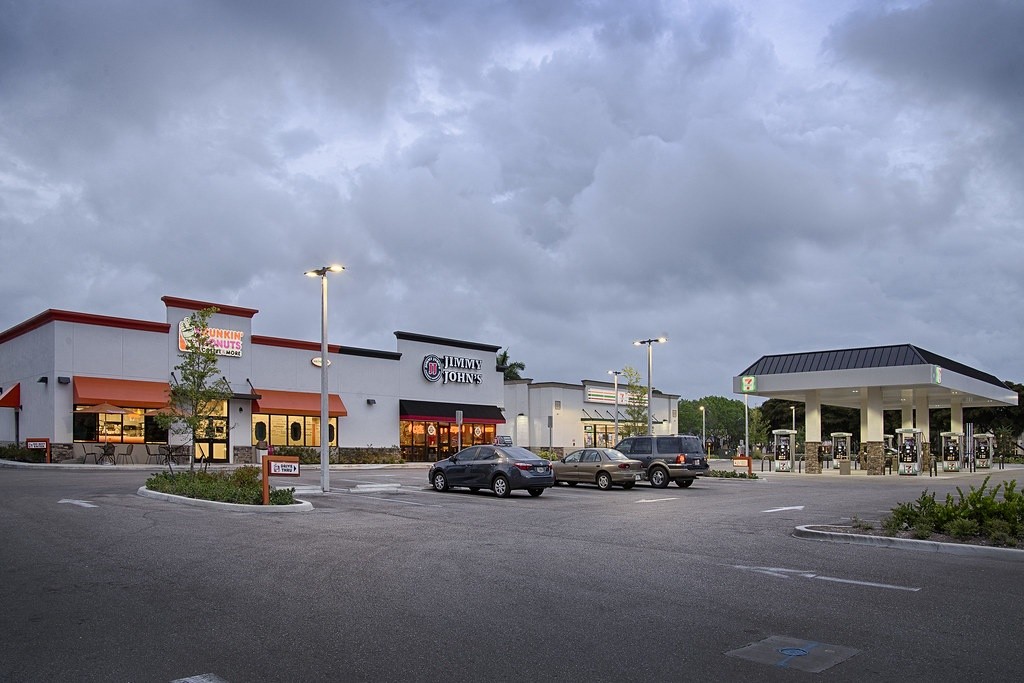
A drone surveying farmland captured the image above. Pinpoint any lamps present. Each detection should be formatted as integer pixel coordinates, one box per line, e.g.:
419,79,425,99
37,376,48,383
58,377,70,385
367,399,376,405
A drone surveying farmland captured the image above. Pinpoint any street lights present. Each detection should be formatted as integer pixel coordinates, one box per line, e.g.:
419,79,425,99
607,371,624,445
700,405,706,449
303,265,346,491
632,337,667,436
789,405,796,430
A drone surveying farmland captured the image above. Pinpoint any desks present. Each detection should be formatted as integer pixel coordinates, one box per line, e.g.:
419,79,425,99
160,446,180,465
94,445,117,465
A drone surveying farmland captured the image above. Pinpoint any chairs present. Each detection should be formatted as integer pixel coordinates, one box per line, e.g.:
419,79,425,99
145,444,159,464
116,444,134,465
158,444,170,465
82,443,98,464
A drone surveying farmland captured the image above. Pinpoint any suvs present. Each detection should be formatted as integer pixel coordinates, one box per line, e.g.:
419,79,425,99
611,433,710,489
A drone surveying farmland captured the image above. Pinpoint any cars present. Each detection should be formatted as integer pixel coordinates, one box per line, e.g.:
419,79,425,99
856,447,903,467
553,448,644,491
428,435,556,498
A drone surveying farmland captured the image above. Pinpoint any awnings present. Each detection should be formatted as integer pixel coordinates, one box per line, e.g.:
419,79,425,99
0,383,20,408
73,376,177,408
399,399,506,424
251,389,348,417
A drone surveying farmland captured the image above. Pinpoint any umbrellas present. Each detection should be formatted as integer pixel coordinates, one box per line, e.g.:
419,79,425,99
140,406,193,462
70,401,135,463
